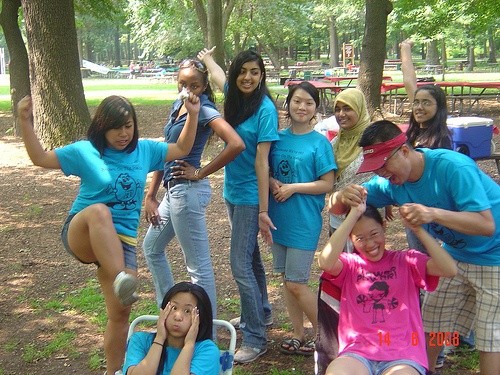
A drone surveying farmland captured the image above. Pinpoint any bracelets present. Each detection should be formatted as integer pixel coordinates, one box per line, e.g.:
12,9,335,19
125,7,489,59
194,167,201,181
151,340,164,349
258,210,269,215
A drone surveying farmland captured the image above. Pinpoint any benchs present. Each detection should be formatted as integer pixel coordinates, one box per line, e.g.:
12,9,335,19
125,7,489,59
106,56,479,77
378,93,408,108
443,94,497,117
391,95,410,114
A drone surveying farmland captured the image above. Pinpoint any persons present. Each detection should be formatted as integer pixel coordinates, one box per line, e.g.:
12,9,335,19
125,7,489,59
326,89,395,254
142,60,245,343
327,119,499,375
197,46,282,363
119,279,222,375
402,85,478,365
15,92,200,375
266,80,341,357
129,60,144,79
316,190,457,374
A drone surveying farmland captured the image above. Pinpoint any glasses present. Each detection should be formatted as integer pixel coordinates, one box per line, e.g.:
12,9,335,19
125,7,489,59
354,232,383,247
412,100,438,107
179,59,207,74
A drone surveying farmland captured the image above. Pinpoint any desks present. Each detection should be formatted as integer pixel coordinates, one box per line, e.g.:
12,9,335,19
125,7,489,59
324,75,394,100
381,80,435,107
435,80,500,115
282,80,343,116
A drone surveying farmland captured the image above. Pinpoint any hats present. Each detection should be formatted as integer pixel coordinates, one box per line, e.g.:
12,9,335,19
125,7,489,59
355,132,408,176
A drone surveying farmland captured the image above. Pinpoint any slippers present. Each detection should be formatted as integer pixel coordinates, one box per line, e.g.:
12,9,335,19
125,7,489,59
280,338,316,355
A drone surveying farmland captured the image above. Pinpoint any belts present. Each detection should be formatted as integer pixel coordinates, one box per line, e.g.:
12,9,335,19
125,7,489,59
164,177,197,190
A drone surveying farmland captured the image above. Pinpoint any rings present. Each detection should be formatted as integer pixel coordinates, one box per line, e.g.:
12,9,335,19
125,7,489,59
181,170,184,175
413,217,417,222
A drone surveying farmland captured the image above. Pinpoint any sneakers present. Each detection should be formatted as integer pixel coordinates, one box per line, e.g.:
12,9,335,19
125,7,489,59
234,346,267,363
230,314,274,329
112,270,140,306
